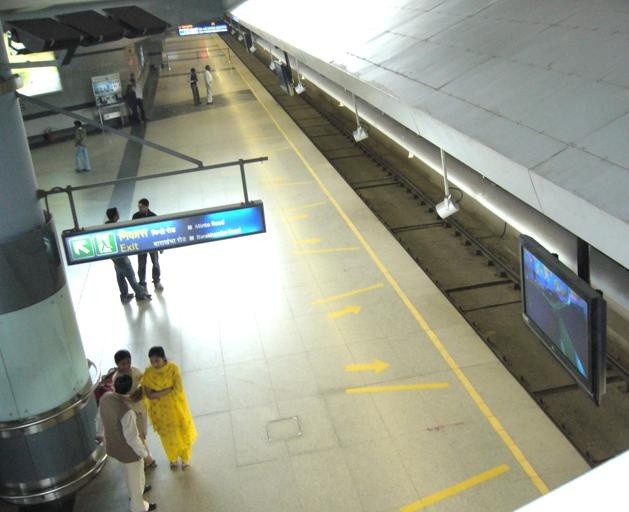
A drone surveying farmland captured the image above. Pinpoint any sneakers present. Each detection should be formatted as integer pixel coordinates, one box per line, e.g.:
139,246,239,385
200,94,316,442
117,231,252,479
119,280,162,302
143,460,194,472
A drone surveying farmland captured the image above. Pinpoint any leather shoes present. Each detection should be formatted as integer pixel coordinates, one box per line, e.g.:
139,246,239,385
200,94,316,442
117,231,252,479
143,485,151,495
148,502,157,512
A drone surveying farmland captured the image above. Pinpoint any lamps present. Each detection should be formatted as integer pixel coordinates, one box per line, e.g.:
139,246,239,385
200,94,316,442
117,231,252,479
431,147,459,220
350,93,369,144
229,27,305,96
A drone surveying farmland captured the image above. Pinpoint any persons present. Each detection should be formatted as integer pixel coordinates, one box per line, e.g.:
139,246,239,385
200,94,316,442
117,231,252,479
139,346,197,471
103,207,151,303
95,374,158,511
113,349,157,469
71,121,92,174
125,84,142,125
131,198,166,290
189,67,202,106
129,79,149,121
205,65,214,104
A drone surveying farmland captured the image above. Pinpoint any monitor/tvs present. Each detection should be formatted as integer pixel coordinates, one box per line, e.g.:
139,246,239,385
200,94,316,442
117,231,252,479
519,233,606,407
274,60,295,97
224,20,252,53
